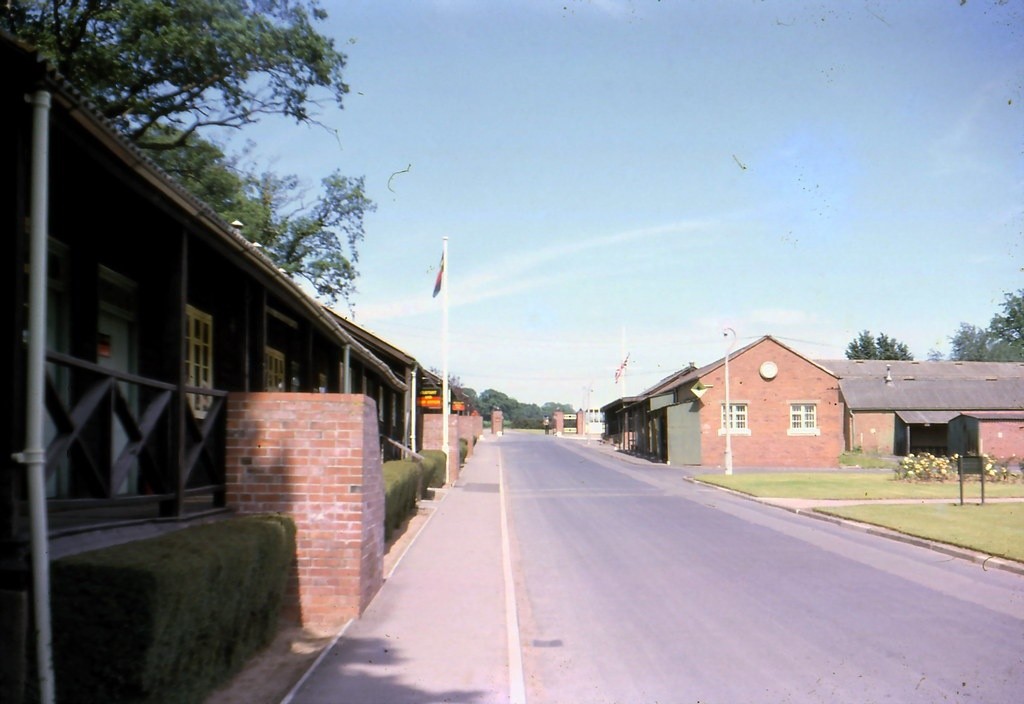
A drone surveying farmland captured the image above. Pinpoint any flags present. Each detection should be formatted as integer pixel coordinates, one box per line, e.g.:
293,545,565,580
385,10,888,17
616,359,627,383
433,252,444,297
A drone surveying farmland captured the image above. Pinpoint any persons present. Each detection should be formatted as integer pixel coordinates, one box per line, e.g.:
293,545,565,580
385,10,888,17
543,415,550,435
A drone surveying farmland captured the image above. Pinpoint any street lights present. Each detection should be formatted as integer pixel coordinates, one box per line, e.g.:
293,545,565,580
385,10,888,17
723,327,736,475
